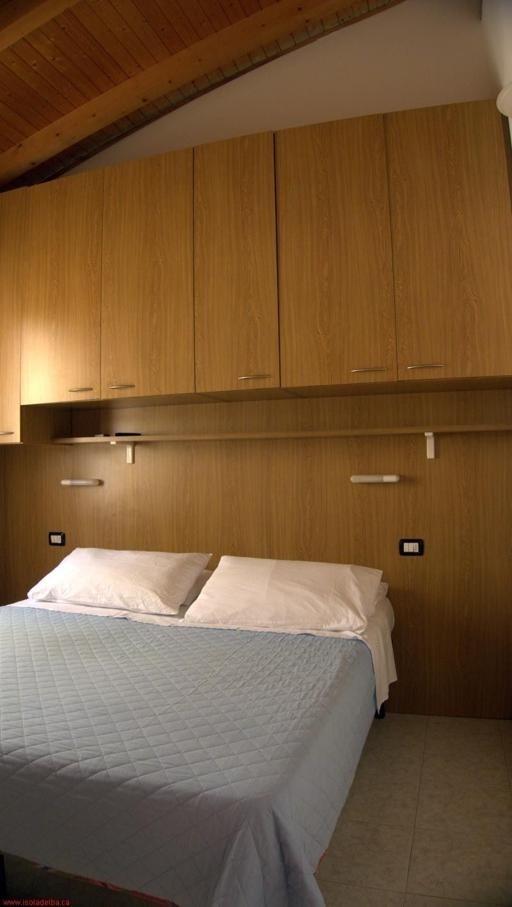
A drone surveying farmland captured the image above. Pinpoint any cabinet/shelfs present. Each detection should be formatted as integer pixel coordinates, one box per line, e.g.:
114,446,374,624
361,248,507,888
23,145,198,410
191,128,279,401
279,95,511,399
0,185,73,447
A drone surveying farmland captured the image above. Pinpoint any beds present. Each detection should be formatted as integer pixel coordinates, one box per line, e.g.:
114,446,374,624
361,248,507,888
0,579,402,907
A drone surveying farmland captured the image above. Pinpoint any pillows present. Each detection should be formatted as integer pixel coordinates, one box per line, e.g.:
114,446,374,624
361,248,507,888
27,547,389,631
